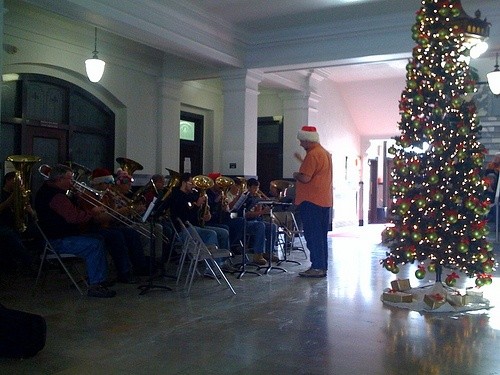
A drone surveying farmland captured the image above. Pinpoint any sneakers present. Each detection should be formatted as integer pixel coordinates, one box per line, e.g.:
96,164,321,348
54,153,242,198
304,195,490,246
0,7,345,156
219,261,237,272
203,267,216,279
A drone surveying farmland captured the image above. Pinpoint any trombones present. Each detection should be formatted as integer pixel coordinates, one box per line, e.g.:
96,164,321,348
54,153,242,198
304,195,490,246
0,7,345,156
37,163,171,244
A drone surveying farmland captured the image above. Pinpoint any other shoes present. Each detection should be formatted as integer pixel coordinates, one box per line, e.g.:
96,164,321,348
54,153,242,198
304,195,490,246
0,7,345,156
127,273,141,283
87,285,116,297
264,254,279,262
298,266,326,277
253,256,267,264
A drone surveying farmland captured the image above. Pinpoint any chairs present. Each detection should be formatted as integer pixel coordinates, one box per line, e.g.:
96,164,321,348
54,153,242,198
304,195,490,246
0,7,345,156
25,180,305,298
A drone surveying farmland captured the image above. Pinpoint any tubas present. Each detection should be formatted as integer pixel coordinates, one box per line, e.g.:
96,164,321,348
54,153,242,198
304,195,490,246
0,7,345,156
7,154,40,233
114,156,296,225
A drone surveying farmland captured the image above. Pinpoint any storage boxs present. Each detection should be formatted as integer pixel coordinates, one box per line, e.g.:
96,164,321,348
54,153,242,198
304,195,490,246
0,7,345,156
424,294,446,309
383,290,413,303
447,286,484,305
391,279,411,292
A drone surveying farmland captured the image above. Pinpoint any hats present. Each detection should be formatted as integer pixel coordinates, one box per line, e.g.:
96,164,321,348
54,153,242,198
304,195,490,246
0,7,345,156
297,126,321,143
87,167,115,185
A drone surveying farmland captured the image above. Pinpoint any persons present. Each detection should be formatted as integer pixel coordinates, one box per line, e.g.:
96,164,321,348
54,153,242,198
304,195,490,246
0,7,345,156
78,167,278,283
293,126,333,278
0,172,50,278
35,165,117,298
